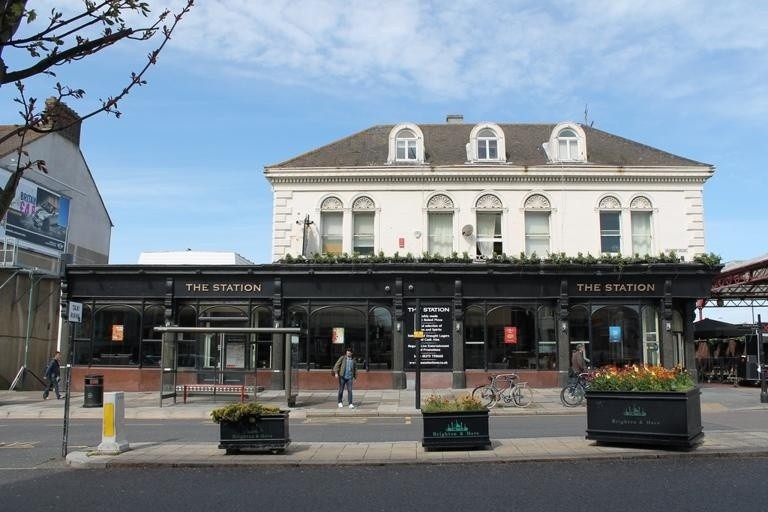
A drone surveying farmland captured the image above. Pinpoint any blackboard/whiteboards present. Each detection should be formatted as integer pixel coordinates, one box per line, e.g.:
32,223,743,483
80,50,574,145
403,305,452,372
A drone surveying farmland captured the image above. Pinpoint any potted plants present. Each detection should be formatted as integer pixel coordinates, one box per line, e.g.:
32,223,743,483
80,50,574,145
211,403,292,453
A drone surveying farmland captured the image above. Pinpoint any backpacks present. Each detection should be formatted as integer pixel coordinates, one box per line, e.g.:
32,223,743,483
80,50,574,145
331,364,341,377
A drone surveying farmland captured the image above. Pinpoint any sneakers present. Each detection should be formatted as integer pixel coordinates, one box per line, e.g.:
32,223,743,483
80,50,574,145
348,403,355,409
338,402,343,408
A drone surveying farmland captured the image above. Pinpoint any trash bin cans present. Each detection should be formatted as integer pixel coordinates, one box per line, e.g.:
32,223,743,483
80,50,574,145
83,374,104,408
737,354,758,379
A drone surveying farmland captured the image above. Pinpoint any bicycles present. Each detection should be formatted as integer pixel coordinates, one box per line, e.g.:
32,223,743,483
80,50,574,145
560,372,589,407
472,374,533,409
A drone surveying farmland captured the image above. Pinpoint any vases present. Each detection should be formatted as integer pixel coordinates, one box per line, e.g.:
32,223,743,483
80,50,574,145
585,387,705,446
422,410,491,447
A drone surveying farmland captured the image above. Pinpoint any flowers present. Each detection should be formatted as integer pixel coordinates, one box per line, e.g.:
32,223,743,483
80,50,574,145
585,360,697,392
420,393,485,410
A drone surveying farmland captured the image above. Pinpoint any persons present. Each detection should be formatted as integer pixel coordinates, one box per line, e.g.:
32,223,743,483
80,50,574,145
568,342,588,398
43,352,67,399
334,348,357,409
